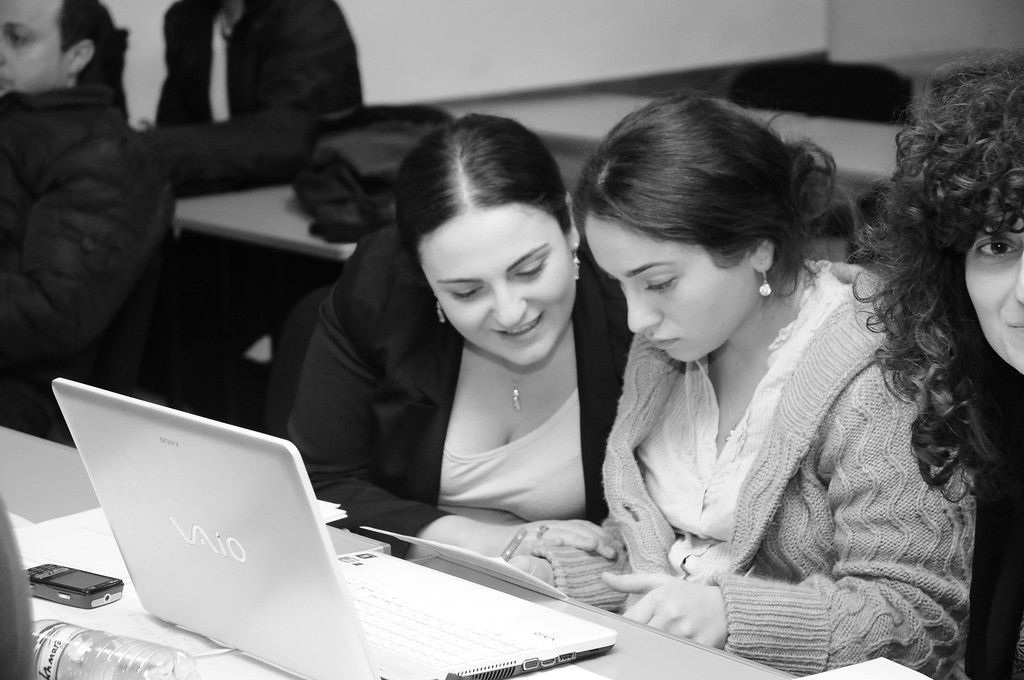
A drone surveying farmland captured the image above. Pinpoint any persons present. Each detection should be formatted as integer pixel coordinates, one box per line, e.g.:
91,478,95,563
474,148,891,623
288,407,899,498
851,55,1023,680
142,0,365,411
287,114,635,560
0,0,174,447
493,91,976,680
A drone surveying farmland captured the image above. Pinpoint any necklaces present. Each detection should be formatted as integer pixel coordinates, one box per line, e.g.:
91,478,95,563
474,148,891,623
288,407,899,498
501,358,533,412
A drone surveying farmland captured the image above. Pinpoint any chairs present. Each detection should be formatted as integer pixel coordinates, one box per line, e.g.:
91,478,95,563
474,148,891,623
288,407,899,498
733,62,916,126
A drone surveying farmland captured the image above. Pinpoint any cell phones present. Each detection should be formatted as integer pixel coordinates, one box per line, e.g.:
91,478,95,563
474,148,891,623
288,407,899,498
22,563,124,610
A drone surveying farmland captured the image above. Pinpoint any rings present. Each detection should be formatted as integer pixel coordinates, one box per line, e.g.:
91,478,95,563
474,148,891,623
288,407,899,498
535,526,548,539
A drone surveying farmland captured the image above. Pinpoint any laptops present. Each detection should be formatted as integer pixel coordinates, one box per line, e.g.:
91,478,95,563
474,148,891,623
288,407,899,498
50,377,617,680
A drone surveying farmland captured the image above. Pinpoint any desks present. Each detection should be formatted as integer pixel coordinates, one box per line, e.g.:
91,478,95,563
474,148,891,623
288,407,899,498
0,430,801,680
166,186,360,261
441,92,905,184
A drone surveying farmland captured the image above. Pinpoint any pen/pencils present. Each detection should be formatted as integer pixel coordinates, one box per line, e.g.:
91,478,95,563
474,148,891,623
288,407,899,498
499,526,529,560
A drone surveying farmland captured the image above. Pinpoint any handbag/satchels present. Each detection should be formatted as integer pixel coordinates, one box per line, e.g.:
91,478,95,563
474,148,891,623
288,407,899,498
300,102,455,243
